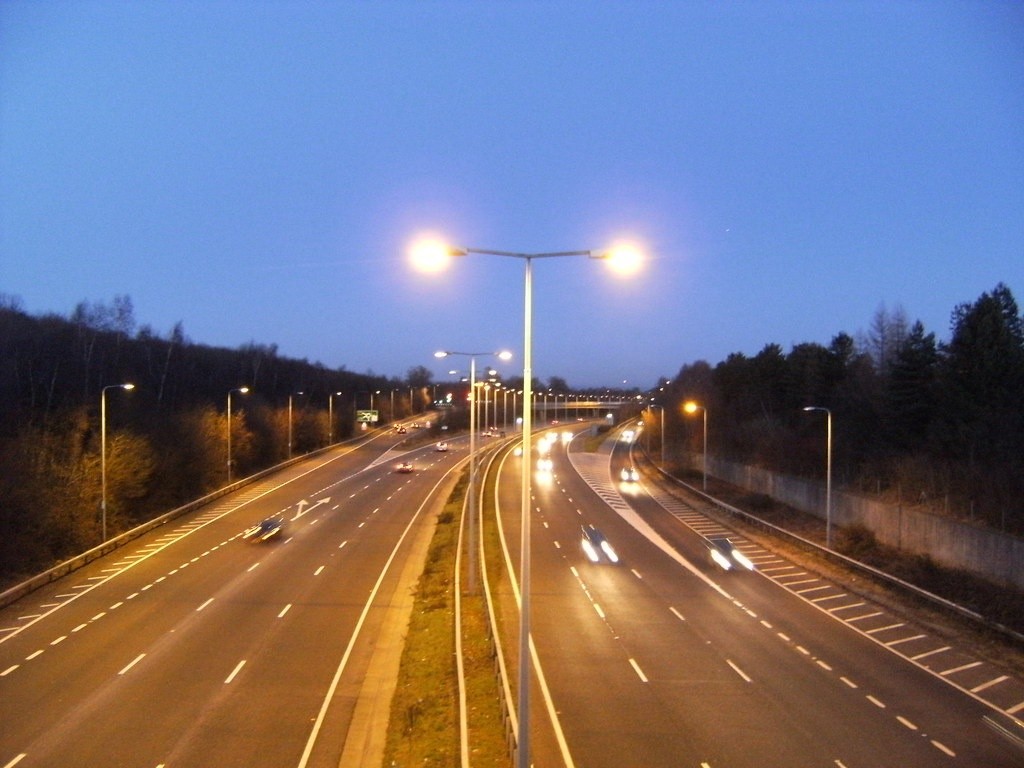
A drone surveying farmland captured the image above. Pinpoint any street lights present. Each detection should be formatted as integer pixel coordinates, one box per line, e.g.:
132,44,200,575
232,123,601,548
802,403,831,547
410,386,419,417
433,348,511,595
102,383,135,540
403,225,651,768
288,391,303,459
228,387,249,485
391,388,400,423
638,402,652,458
650,402,665,469
371,390,381,430
447,367,640,484
328,391,342,447
687,401,709,493
